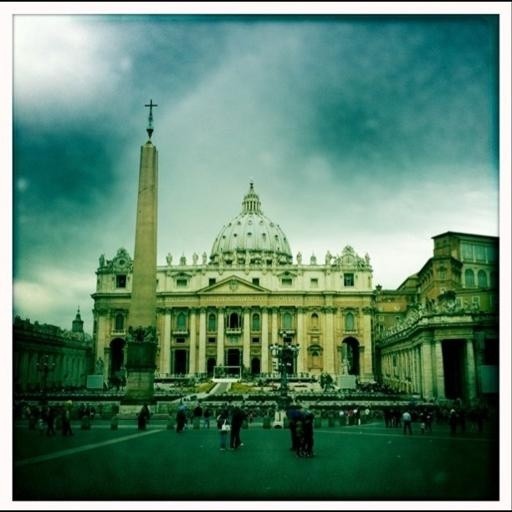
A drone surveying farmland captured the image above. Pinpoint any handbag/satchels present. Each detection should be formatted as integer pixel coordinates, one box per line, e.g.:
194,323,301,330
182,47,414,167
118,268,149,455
222,424,231,431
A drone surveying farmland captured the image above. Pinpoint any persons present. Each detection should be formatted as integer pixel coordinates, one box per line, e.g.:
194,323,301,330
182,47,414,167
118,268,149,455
137,403,151,432
175,403,210,434
286,403,314,457
17,403,96,437
346,404,362,427
215,407,244,451
384,404,487,434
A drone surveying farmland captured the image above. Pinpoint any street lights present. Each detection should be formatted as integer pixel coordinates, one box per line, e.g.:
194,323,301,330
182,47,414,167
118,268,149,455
269,332,300,396
36,354,56,406
